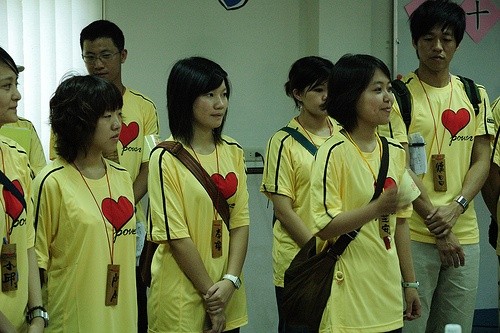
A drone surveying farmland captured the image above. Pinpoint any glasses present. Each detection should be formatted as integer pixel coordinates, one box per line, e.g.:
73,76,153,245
82,49,123,62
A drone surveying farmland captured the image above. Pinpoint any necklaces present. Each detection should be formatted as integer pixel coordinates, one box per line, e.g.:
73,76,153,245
295,116,331,148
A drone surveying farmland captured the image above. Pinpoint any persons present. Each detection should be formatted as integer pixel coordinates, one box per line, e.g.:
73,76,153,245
259,56,342,333
49,17,157,333
149,56,249,333
0,65,48,175
0,46,48,333
482,93,500,301
316,54,423,333
33,76,137,333
381,0,490,333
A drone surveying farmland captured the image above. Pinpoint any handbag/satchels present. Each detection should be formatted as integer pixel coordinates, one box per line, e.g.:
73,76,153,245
138,238,159,287
283,235,338,328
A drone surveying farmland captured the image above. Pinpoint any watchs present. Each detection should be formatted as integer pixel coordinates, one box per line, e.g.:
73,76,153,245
402,281,420,289
29,311,49,327
454,195,469,214
222,274,243,290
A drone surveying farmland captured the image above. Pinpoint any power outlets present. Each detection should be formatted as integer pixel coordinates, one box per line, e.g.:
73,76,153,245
244,147,263,162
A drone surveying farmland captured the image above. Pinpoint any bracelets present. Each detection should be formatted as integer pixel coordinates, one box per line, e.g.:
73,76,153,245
26,306,46,322
